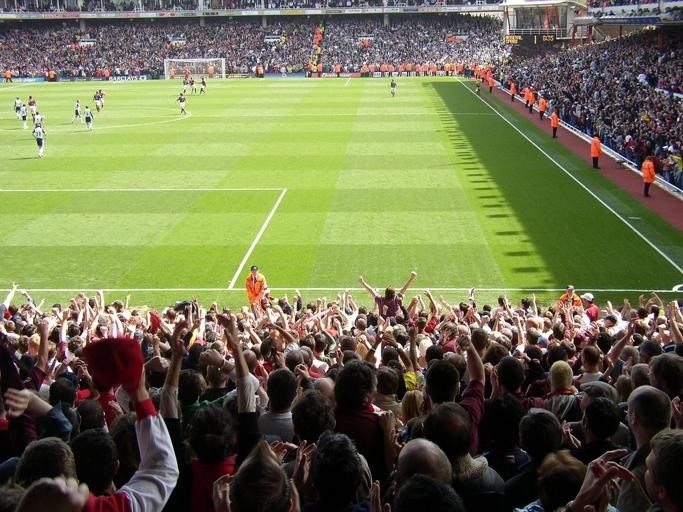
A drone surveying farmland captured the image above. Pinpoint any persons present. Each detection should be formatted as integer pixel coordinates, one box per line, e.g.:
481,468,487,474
175,76,206,115
358,271,416,318
498,0,683,197
1,0,499,79
391,79,398,96
476,78,494,95
1,280,682,512
245,266,267,309
72,89,106,130
13,95,46,129
31,123,45,158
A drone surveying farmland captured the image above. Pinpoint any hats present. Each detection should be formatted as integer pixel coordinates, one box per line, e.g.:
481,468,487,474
580,293,594,302
580,380,617,400
